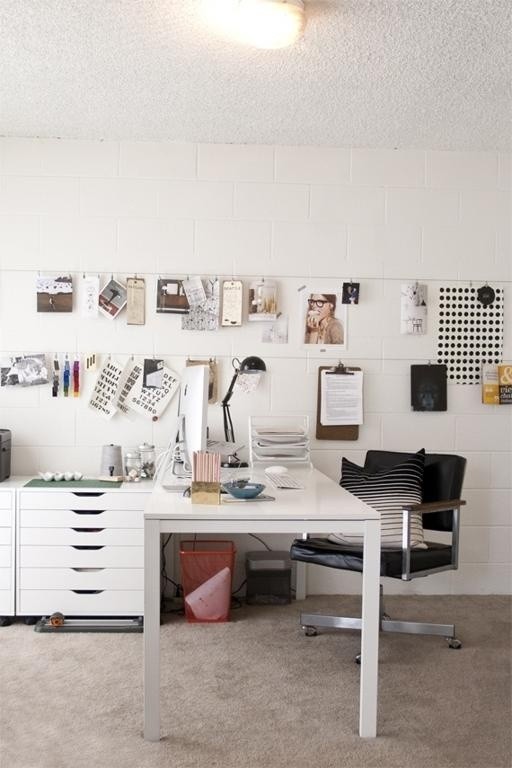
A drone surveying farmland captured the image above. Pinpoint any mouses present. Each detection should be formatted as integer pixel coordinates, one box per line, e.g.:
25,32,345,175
264,466,289,474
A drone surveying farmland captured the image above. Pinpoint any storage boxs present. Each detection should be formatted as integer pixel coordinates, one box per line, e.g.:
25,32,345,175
0,429,11,481
246,551,291,604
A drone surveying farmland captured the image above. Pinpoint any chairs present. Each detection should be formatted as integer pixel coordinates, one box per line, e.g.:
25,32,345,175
288,450,467,663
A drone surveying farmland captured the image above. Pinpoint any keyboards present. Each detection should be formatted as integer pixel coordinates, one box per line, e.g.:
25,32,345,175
264,472,305,489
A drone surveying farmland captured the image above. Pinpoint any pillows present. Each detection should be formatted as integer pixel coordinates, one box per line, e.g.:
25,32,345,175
329,448,430,552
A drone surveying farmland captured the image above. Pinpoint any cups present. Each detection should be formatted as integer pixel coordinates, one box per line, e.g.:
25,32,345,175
122,452,142,483
138,442,158,481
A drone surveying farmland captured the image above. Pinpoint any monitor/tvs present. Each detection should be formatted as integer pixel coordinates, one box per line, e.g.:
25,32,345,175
155,364,211,494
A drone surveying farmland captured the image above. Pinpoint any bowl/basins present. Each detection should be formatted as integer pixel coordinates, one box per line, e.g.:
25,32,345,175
38,471,83,483
222,481,267,499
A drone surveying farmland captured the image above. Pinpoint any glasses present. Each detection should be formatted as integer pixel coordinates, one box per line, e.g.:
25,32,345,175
308,299,330,307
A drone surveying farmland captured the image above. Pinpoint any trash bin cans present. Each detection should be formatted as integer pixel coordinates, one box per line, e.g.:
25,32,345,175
179,540,237,623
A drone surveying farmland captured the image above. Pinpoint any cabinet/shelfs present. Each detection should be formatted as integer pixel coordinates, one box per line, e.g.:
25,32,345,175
0,489,158,625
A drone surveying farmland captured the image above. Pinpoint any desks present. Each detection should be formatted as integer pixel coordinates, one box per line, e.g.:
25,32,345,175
143,454,380,742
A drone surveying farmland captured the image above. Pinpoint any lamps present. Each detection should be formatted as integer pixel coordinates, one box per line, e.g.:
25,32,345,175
221,356,266,469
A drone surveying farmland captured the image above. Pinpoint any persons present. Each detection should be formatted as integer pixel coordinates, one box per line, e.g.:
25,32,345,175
306,295,344,344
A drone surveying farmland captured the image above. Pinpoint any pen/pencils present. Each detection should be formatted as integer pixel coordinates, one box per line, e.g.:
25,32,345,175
193,450,222,482
